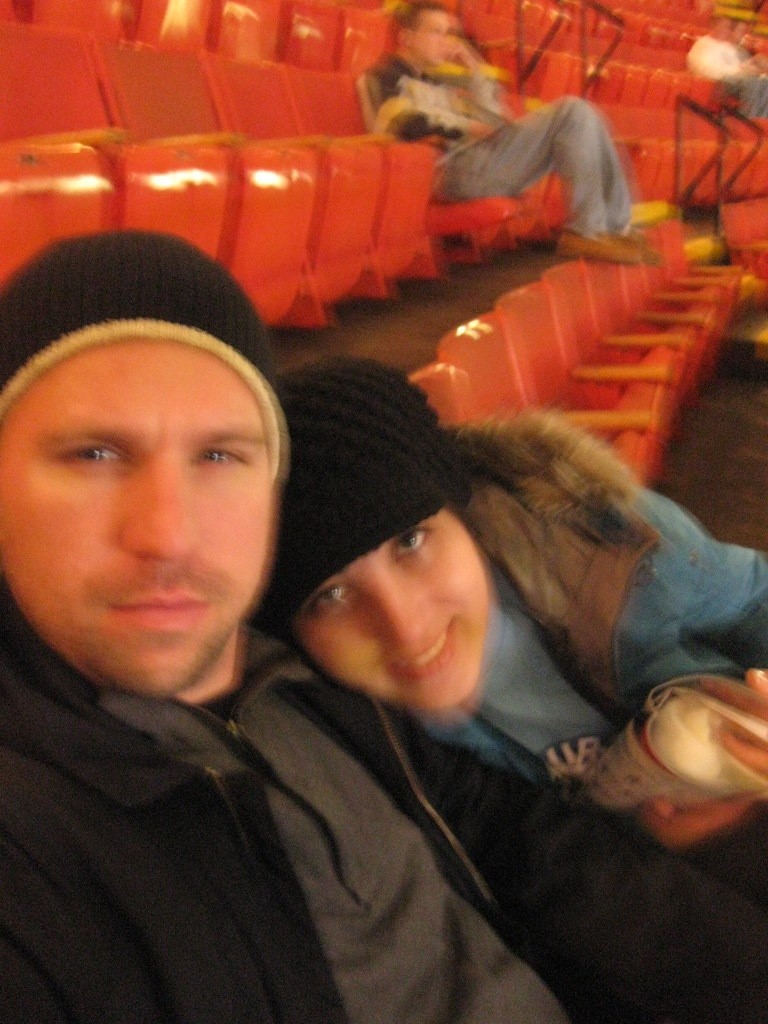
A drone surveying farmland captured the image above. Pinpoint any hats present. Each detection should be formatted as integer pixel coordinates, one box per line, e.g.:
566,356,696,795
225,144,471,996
0,226,292,486
247,354,475,659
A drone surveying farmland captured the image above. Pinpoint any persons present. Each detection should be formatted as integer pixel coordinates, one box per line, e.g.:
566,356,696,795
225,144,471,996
688,14,768,118
2,229,767,1024
358,0,657,268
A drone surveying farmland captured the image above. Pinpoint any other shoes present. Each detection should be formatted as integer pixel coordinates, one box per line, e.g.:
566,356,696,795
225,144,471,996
554,229,663,267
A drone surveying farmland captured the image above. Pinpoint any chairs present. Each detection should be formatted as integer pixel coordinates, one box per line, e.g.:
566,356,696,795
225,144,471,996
0,0,768,494
